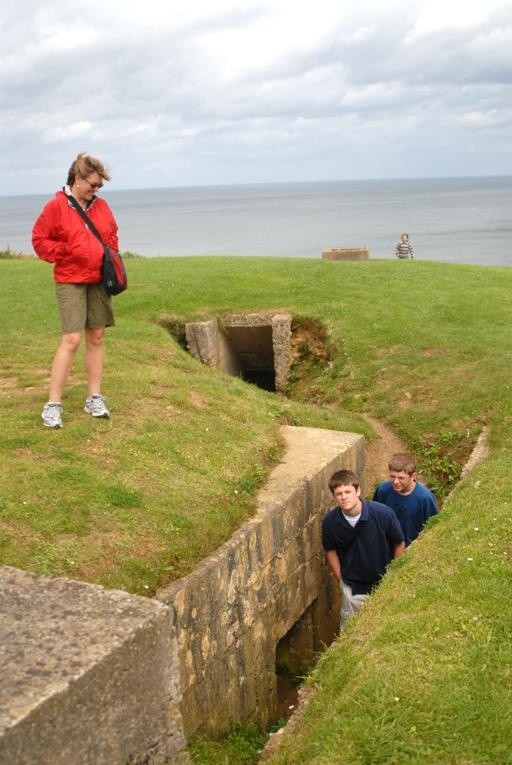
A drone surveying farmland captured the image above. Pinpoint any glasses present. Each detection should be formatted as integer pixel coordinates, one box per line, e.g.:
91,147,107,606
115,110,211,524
82,177,104,189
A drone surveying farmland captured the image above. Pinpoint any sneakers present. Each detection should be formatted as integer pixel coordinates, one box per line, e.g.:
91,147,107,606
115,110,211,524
39,401,64,430
83,393,112,419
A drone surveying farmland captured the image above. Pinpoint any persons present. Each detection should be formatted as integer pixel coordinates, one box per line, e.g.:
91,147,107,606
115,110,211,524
323,454,440,634
395,231,414,260
31,154,128,429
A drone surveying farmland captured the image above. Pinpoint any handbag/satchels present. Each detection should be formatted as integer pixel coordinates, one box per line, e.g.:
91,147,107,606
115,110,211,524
101,247,128,298
